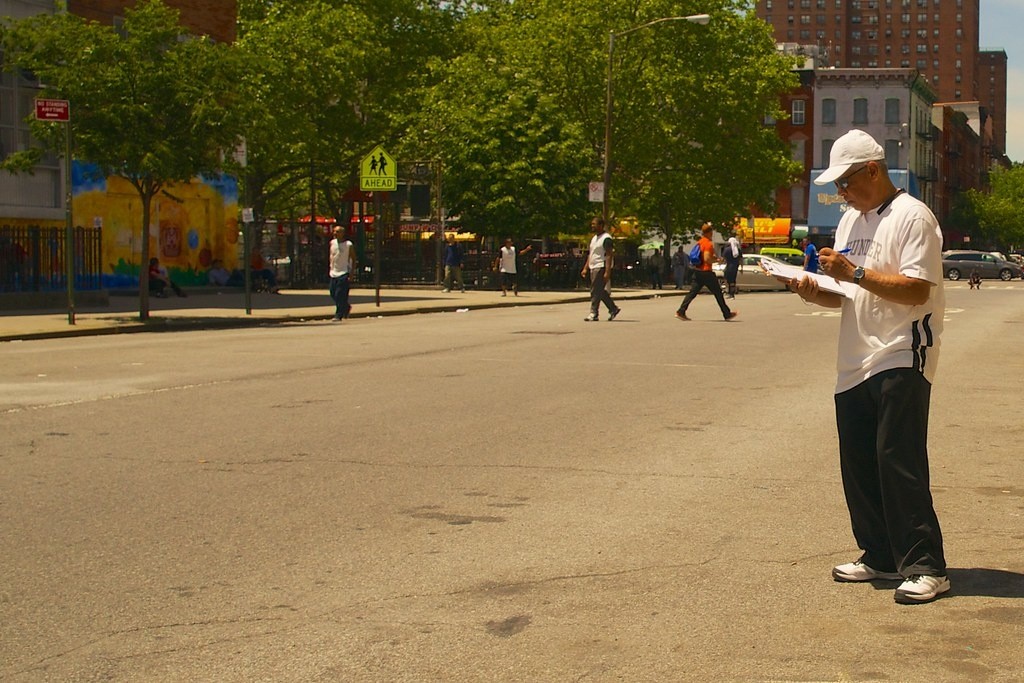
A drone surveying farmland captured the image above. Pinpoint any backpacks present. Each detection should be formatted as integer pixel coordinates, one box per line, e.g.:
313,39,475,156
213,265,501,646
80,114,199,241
690,244,701,265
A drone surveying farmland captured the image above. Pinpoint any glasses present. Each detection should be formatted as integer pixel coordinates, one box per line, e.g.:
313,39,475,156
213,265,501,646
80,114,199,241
834,165,867,189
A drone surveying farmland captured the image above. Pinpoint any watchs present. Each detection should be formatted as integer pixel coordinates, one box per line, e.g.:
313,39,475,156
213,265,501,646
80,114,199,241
854,266,865,284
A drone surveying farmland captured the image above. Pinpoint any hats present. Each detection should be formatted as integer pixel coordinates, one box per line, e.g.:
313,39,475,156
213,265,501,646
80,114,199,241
702,223,712,233
814,130,885,185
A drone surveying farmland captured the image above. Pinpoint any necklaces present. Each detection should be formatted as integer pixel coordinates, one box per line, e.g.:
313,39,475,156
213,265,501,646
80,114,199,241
877,188,905,215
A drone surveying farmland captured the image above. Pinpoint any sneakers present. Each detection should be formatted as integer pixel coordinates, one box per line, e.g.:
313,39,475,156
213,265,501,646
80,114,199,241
894,574,951,603
832,557,904,580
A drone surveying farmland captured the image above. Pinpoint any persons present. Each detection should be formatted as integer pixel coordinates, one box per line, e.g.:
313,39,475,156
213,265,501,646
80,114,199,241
798,237,817,273
673,246,692,289
581,216,621,321
493,238,532,296
207,247,276,287
675,224,738,321
648,249,666,289
777,129,951,604
968,268,982,289
442,234,465,293
329,226,355,322
720,237,743,300
139,257,187,298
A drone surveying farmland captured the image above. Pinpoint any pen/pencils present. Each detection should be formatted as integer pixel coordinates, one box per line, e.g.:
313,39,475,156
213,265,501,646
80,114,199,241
815,248,851,258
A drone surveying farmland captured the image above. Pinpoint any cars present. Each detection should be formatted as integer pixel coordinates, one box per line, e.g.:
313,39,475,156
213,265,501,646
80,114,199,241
1009,254,1023,263
711,254,804,293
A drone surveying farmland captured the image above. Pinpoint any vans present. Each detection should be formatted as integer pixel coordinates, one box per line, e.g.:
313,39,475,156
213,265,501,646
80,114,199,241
941,250,1022,282
761,248,804,259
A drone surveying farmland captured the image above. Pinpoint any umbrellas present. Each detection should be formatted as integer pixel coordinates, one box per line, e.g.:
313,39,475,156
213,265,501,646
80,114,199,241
637,240,665,253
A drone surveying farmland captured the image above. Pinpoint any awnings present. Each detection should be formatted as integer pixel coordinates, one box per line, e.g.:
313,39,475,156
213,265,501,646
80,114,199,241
401,233,481,240
791,230,808,239
702,217,791,244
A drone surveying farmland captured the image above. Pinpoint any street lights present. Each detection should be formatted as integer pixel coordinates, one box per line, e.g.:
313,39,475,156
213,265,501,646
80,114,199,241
603,14,710,295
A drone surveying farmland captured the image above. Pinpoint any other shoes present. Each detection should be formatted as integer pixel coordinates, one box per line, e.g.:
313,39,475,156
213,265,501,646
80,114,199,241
725,313,735,320
584,313,598,321
608,306,620,320
675,311,691,321
